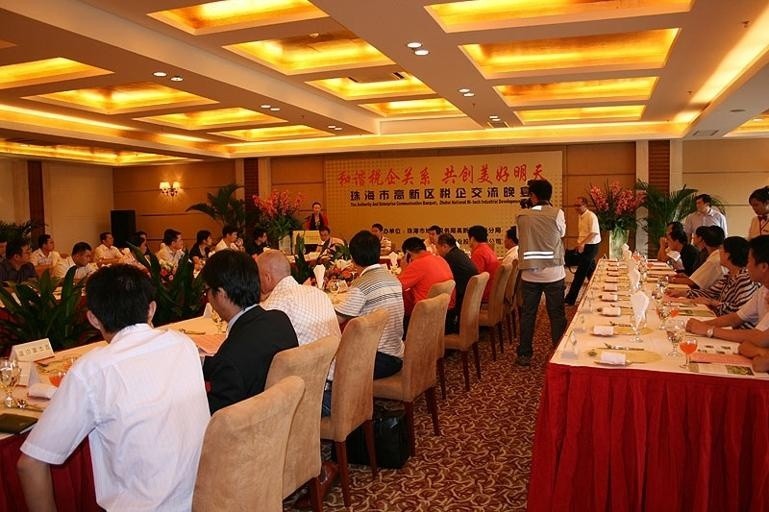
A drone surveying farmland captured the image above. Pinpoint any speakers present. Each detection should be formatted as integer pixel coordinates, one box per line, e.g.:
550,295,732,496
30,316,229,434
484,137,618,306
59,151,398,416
110,210,136,248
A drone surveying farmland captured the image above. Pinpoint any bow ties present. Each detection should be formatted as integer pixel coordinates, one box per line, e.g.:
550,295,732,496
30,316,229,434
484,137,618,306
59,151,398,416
758,215,768,222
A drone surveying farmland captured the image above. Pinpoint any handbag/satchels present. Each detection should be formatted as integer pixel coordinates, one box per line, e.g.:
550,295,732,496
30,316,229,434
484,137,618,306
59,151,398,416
563,246,583,274
345,404,413,470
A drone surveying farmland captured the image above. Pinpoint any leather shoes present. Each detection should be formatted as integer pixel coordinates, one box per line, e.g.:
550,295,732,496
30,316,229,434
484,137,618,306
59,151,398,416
515,356,531,366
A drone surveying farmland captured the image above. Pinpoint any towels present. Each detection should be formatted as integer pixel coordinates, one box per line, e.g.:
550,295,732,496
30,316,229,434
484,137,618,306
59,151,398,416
608,272,618,275
593,326,614,336
602,306,621,316
604,284,617,291
610,262,619,266
602,294,617,301
599,351,626,366
608,267,617,270
609,258,618,261
606,277,618,282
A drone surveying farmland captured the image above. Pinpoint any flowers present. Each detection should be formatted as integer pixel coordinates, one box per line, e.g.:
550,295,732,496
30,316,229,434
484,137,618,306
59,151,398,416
581,179,650,244
185,182,260,257
0,265,102,352
291,233,331,283
126,242,208,326
0,220,50,249
251,190,305,257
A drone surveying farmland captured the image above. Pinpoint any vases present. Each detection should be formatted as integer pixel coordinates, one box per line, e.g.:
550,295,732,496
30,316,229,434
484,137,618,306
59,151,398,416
278,235,291,256
608,230,629,258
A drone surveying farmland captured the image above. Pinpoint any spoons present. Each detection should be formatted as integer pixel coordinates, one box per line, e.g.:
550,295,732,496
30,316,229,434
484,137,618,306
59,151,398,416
17,399,46,411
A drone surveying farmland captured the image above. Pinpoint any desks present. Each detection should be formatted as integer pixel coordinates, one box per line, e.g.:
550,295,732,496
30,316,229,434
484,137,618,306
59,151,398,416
0,286,101,349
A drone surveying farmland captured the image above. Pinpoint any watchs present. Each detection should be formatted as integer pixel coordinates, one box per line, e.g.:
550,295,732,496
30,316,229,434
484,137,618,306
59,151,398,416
706,325,714,337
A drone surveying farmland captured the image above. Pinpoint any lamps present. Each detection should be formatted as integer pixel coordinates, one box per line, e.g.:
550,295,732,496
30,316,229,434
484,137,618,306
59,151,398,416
407,43,422,48
153,72,168,76
489,116,498,118
334,128,341,130
464,93,475,97
415,50,429,55
160,181,181,198
271,108,280,111
459,88,470,93
261,105,270,109
171,77,182,81
492,119,500,121
328,126,336,128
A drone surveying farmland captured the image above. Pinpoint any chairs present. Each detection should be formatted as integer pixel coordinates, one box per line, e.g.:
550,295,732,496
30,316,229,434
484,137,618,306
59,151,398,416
428,280,457,413
373,292,450,456
480,263,513,362
321,309,390,506
265,334,343,511
504,259,521,346
444,272,490,390
193,377,306,512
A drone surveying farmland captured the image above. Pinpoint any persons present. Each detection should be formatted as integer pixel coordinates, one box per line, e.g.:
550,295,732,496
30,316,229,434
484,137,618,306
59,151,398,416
435,233,480,335
313,228,340,252
564,196,603,307
156,228,187,272
501,229,520,305
216,224,241,252
664,226,708,276
333,232,404,433
54,242,98,285
118,235,150,268
668,225,731,289
397,237,456,336
138,231,157,264
245,229,274,256
254,249,342,503
746,185,769,242
424,226,442,255
18,265,212,511
372,223,392,256
93,233,123,268
468,225,502,309
667,230,699,276
686,234,769,348
517,179,569,364
203,249,299,418
29,234,63,271
663,235,763,330
304,203,331,230
680,193,729,252
0,238,40,288
190,230,214,269
657,223,682,264
0,237,8,263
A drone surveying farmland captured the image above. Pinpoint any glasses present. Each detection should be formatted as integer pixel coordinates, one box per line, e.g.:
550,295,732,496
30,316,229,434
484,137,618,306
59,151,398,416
200,286,211,297
574,203,584,207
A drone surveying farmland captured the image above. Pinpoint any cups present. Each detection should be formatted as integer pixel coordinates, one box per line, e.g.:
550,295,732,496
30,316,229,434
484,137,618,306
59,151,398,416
390,265,397,274
640,272,648,290
655,299,671,331
316,279,324,291
664,317,685,359
330,278,340,302
656,274,669,299
63,353,82,373
48,361,65,387
628,311,647,344
211,309,224,336
670,302,680,318
0,360,22,409
464,247,470,258
679,335,698,373
